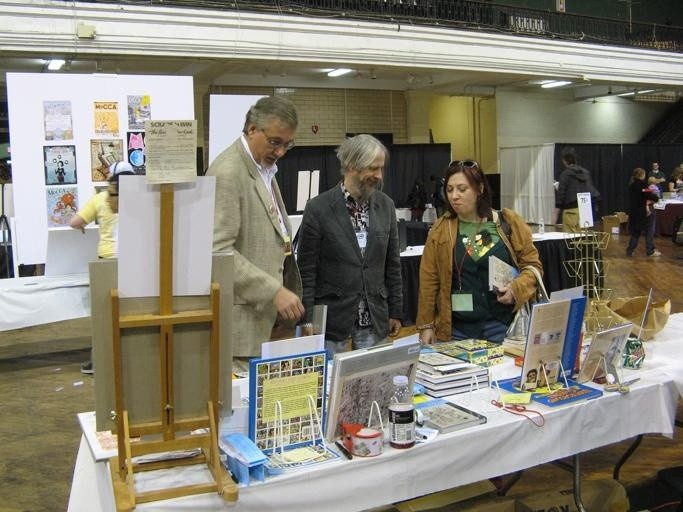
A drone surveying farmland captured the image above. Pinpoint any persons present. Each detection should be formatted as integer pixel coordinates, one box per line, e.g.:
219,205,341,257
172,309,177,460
69,161,137,374
642,176,662,216
549,146,603,233
647,161,665,197
202,95,306,378
106,171,142,260
297,135,405,361
625,168,662,259
415,159,544,346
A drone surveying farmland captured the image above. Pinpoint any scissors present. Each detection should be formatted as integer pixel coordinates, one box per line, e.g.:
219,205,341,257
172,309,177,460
604,378,640,393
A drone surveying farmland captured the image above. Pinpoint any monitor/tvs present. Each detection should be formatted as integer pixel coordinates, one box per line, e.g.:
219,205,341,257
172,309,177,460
384,143,451,227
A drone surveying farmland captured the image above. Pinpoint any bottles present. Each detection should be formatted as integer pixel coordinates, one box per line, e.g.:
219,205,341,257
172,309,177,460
621,338,644,369
539,217,544,236
389,376,415,448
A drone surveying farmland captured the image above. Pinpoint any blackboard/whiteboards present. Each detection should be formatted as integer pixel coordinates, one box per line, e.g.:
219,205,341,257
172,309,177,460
206,93,270,167
4,72,194,266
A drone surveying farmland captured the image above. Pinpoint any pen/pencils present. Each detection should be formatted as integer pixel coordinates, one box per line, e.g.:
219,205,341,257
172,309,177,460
335,441,353,460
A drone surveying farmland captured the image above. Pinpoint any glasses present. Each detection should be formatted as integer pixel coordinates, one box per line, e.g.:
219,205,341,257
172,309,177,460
449,160,479,170
262,128,296,151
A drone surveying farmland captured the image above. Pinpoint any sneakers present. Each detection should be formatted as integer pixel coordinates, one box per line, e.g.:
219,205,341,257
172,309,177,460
81,361,95,374
628,250,639,257
648,251,662,256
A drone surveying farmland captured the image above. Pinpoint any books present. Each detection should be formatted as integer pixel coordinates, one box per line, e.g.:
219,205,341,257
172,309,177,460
419,347,490,398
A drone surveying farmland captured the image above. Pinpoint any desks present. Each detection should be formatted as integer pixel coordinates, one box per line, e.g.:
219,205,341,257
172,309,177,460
312,230,602,325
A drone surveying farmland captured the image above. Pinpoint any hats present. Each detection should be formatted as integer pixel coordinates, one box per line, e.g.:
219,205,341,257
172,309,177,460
106,160,135,179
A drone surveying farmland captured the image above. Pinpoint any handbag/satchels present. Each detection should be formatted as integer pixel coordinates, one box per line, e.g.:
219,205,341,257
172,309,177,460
507,265,550,336
